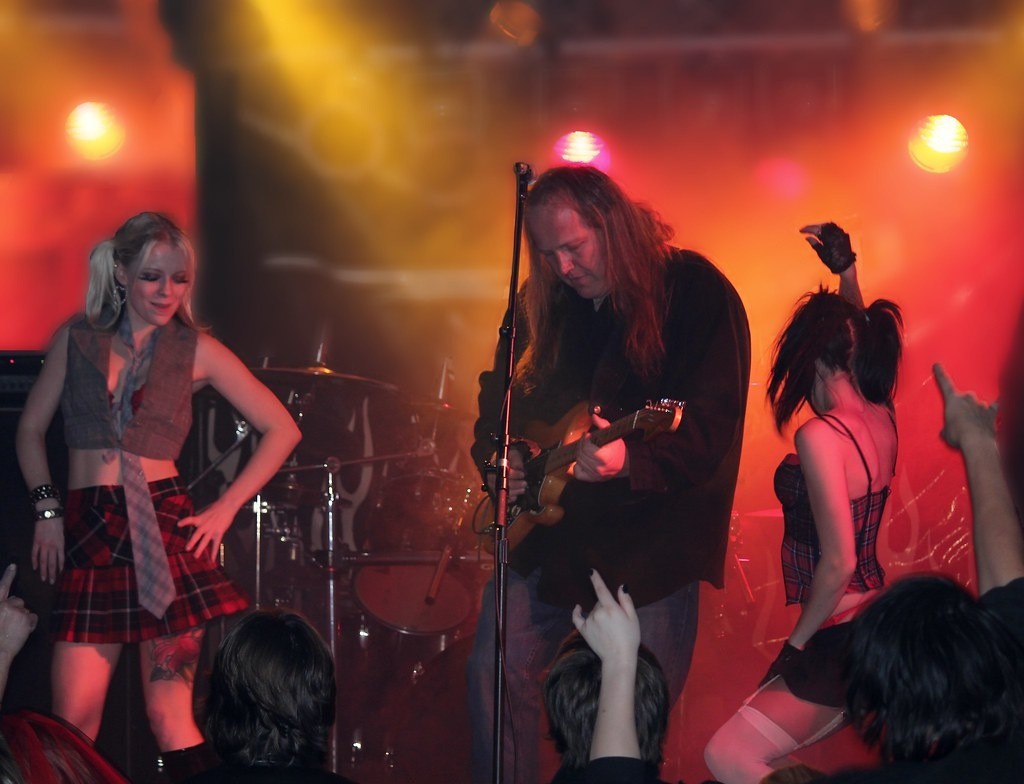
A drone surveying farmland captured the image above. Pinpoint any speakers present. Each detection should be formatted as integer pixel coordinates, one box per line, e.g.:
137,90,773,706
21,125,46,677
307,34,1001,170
0,351,202,784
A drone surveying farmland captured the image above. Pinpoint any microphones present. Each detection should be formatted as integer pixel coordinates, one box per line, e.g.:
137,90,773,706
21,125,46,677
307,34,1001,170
515,161,538,183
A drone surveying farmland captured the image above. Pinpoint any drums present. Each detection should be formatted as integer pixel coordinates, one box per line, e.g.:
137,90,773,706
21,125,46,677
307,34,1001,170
378,470,488,562
202,501,308,611
355,557,477,665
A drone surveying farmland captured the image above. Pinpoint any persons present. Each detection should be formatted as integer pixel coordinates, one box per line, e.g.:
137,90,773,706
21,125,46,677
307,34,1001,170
0,564,38,708
15,211,303,775
465,164,752,784
0,712,127,784
841,362,1024,784
541,630,669,784
170,609,357,784
572,568,641,784
703,222,904,784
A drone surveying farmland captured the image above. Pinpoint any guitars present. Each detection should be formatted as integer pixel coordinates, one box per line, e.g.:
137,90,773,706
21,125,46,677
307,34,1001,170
481,394,687,560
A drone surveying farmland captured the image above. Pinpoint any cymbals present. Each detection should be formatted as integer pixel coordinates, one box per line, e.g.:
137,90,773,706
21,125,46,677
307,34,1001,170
412,401,472,417
249,363,400,395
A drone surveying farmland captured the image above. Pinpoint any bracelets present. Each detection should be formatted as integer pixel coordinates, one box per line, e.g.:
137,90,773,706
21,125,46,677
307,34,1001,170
33,508,63,522
28,484,61,512
